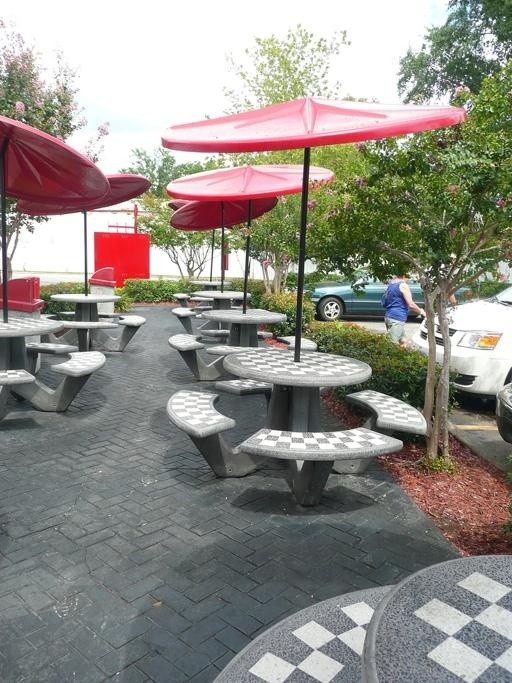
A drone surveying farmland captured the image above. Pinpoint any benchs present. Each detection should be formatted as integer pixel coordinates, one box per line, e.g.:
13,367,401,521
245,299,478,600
206,345,264,377
216,378,326,412
48,349,107,412
172,306,197,334
277,335,318,351
1,369,36,403
333,389,429,474
173,278,251,311
169,333,208,382
40,312,58,343
166,389,236,477
58,310,121,319
240,425,404,505
213,582,396,682
63,319,118,351
200,329,273,343
119,315,146,354
24,343,79,373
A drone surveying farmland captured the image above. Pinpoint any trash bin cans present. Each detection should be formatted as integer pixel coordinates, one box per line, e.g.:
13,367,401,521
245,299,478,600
0,276,46,374
88,267,116,322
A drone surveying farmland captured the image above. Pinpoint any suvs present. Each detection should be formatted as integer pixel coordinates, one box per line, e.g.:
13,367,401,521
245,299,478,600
413,285,512,410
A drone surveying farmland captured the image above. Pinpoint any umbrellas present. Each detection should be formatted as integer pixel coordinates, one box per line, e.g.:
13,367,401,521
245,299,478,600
0,114,112,325
169,196,278,292
167,164,335,315
15,173,151,296
167,199,215,281
160,91,471,363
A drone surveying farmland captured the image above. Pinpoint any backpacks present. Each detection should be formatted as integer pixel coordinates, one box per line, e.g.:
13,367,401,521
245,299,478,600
381,291,393,308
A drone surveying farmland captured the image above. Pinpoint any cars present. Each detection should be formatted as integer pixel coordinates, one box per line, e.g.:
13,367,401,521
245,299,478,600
302,267,474,320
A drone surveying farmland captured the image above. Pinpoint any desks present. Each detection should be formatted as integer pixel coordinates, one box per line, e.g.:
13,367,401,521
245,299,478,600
50,293,121,352
363,553,512,681
1,317,63,411
223,349,372,461
201,308,287,347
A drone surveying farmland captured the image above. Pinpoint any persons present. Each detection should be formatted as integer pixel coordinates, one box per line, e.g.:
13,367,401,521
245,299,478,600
380,271,426,349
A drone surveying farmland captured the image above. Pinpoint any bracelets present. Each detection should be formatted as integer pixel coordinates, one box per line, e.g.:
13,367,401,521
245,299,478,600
419,308,424,314
